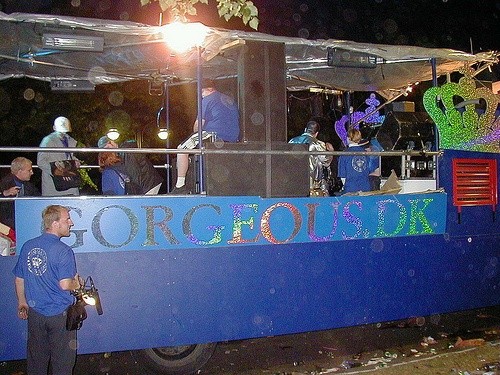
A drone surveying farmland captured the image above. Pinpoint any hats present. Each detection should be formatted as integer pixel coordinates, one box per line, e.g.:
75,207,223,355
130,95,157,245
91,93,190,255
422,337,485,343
196,78,215,89
53,116,73,132
98,135,112,147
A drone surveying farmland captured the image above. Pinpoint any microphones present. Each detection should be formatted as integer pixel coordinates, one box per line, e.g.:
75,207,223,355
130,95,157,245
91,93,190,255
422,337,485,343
89,278,103,315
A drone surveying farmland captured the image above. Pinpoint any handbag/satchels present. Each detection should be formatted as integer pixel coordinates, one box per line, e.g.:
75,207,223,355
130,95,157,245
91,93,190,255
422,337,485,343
50,159,84,191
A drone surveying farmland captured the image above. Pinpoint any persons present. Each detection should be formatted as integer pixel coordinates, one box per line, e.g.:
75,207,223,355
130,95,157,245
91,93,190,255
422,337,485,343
11,204,87,375
37,116,81,195
97,143,164,196
337,128,380,196
167,78,241,195
0,156,40,235
98,136,134,196
287,120,335,198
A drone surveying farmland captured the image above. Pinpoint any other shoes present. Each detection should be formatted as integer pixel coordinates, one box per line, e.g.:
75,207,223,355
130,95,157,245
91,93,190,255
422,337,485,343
170,184,186,195
